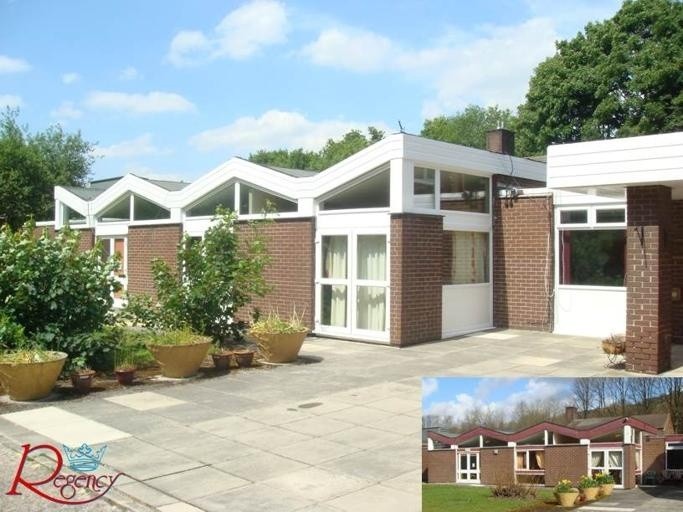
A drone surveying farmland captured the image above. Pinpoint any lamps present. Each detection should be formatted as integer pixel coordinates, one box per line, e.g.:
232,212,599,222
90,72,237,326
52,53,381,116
671,287,681,302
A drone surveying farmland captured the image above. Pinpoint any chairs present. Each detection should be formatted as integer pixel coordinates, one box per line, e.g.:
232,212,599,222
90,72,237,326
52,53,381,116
644,470,656,484
658,470,675,484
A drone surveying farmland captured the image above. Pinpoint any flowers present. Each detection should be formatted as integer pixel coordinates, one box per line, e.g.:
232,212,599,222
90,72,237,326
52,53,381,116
552,479,574,492
594,472,615,484
576,475,599,488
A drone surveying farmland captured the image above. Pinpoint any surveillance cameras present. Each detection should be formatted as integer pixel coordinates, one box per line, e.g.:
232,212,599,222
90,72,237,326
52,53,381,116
462,191,470,200
511,187,518,197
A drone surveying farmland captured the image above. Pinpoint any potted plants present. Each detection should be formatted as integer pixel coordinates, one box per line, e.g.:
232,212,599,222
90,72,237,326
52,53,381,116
248,300,312,363
234,344,254,369
114,348,137,385
70,365,96,396
208,343,232,370
144,319,214,379
0,341,69,401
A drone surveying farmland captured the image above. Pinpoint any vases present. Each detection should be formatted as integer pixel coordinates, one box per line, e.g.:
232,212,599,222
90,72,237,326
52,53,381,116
598,484,613,497
603,335,627,354
553,492,579,508
579,488,599,502
